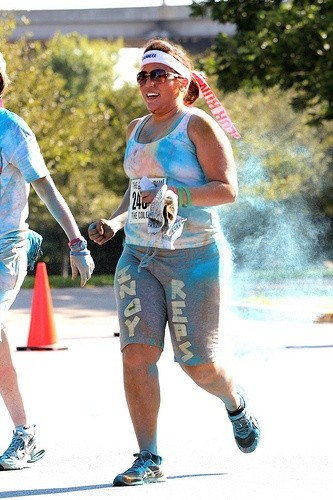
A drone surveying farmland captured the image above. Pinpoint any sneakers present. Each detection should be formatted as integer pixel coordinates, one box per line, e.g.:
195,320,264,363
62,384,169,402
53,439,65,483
112,449,167,487
0,424,46,470
224,391,260,453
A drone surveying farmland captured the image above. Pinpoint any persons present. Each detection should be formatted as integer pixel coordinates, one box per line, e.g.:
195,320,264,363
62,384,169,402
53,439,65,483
0,48,96,471
88,39,261,486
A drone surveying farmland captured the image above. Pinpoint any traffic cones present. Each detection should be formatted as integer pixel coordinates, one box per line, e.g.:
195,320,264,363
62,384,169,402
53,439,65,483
16,262,68,352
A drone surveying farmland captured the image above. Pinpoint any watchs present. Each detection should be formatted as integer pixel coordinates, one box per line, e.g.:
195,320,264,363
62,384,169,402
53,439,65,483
69,240,88,251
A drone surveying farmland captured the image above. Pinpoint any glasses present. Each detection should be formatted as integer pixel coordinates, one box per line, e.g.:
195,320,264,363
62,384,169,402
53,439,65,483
136,69,182,86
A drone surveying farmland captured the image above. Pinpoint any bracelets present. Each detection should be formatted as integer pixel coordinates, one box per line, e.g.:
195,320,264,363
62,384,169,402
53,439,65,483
175,184,193,211
69,250,91,256
67,236,87,246
